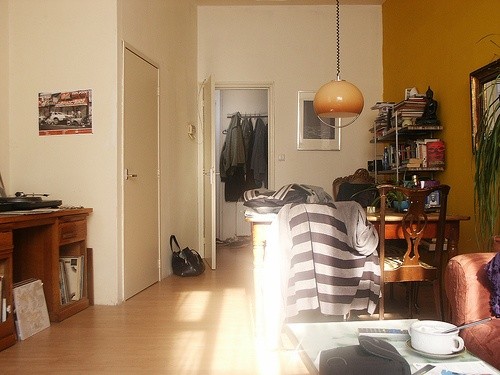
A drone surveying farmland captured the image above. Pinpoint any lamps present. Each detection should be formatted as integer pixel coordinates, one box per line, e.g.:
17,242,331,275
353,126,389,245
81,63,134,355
313,0,364,127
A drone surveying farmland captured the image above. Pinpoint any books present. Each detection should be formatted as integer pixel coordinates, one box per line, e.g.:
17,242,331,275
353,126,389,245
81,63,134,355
374,97,428,137
390,137,445,168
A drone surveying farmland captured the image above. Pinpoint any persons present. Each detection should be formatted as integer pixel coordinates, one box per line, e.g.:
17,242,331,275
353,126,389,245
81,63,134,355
415,85,439,124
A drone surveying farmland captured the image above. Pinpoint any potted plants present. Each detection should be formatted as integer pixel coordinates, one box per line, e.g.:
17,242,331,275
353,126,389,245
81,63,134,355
473,32,500,251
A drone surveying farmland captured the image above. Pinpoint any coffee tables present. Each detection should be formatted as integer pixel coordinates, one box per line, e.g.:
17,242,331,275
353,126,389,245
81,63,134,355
281,319,500,375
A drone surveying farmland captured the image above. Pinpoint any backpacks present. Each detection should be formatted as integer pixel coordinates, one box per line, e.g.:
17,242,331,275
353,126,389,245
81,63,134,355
319,335,412,375
243,181,333,213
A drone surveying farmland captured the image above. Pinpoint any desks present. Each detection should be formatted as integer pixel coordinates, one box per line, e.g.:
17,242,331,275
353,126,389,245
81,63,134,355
246,207,470,319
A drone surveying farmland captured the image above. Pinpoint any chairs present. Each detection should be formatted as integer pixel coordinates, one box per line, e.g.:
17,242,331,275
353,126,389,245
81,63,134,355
375,182,451,320
332,167,374,202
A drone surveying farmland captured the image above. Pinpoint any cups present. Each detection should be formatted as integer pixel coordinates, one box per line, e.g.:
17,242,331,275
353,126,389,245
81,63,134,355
407,320,464,354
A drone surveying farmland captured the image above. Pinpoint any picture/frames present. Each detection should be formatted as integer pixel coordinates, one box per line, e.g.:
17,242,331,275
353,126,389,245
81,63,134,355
297,90,341,151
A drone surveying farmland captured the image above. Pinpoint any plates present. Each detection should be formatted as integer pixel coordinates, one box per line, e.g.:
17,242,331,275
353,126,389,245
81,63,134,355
404,338,466,360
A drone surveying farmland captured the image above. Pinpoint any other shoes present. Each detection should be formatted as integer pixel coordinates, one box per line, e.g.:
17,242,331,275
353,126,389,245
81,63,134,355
216,238,230,246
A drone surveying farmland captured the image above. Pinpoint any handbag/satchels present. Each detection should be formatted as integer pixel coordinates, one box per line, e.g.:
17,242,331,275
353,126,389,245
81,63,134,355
169,235,206,277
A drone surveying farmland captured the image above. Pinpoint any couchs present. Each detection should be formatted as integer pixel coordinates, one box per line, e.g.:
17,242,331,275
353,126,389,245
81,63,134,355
444,251,500,373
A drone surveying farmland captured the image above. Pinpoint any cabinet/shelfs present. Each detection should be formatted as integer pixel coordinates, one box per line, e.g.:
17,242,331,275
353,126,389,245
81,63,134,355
370,110,443,185
0,207,95,350
